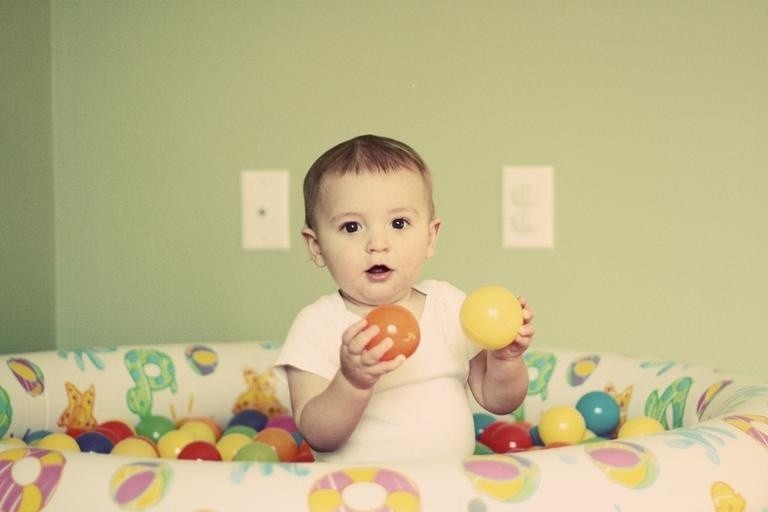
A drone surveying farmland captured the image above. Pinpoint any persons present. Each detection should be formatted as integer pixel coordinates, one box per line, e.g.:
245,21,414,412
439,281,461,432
264,131,535,465
